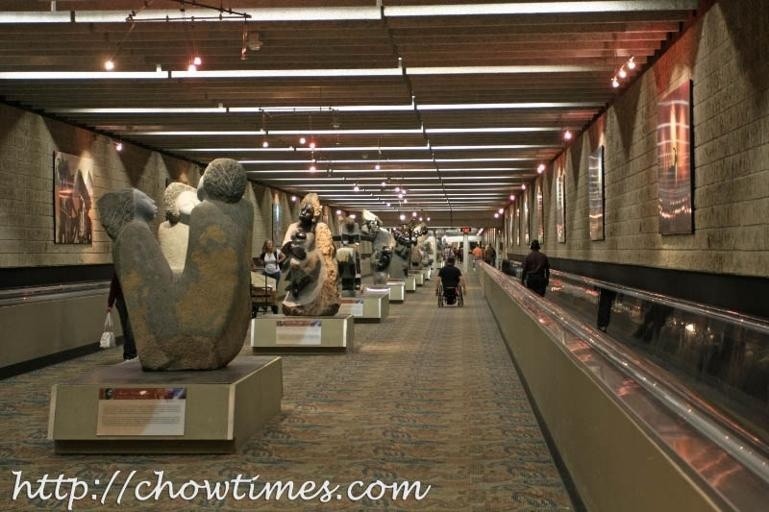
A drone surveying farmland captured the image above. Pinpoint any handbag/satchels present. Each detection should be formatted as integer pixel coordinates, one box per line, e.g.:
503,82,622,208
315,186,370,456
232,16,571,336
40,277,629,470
99,311,116,348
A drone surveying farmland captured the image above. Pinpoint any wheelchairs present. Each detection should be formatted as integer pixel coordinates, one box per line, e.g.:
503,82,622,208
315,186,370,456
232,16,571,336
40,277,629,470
437,283,463,308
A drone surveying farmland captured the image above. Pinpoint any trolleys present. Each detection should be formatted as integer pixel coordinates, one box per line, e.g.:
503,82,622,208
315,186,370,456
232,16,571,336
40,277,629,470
250,268,278,318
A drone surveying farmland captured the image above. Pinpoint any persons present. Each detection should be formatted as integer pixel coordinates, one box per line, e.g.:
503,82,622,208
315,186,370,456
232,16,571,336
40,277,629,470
443,246,463,264
472,243,496,267
520,239,550,298
435,257,466,305
